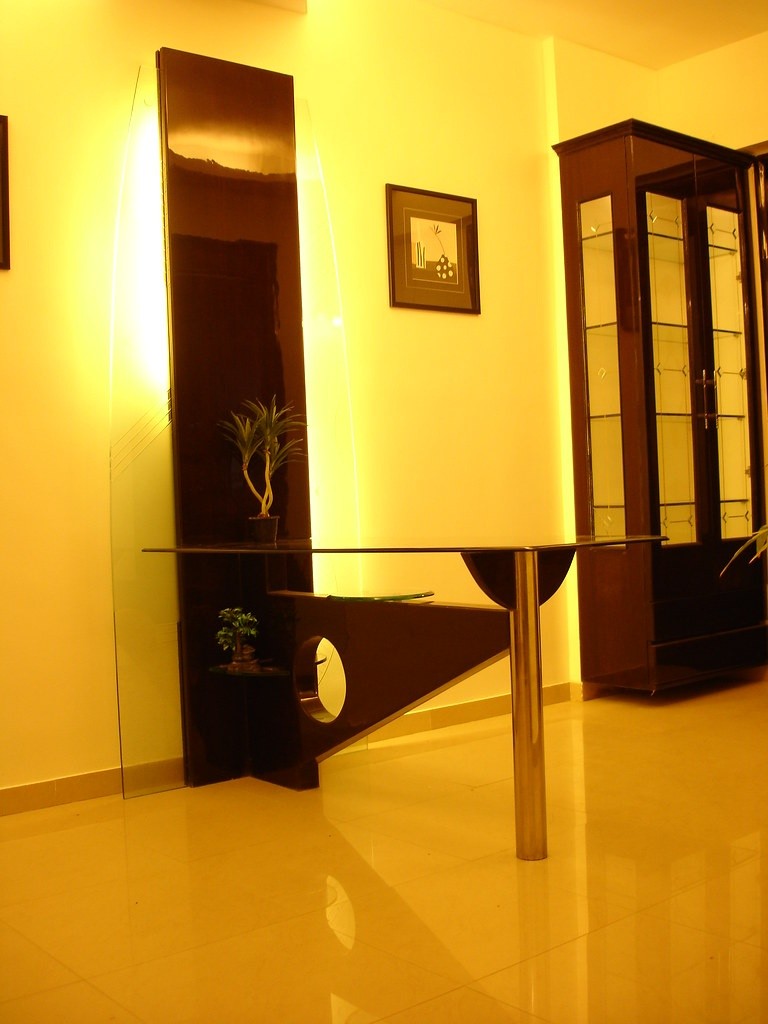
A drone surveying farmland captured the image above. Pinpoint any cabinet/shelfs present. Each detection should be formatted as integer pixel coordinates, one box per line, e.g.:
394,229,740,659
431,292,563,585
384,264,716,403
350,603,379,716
552,117,768,701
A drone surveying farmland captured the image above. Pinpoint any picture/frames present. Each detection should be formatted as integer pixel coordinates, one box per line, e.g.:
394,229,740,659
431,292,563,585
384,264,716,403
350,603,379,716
384,182,482,315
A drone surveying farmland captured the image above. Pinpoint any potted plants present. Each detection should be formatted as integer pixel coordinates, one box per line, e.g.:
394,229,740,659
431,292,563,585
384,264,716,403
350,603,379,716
196,603,298,774
215,392,311,543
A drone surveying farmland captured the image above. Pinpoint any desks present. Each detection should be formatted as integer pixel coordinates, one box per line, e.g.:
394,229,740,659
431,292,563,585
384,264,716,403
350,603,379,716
139,534,670,863
